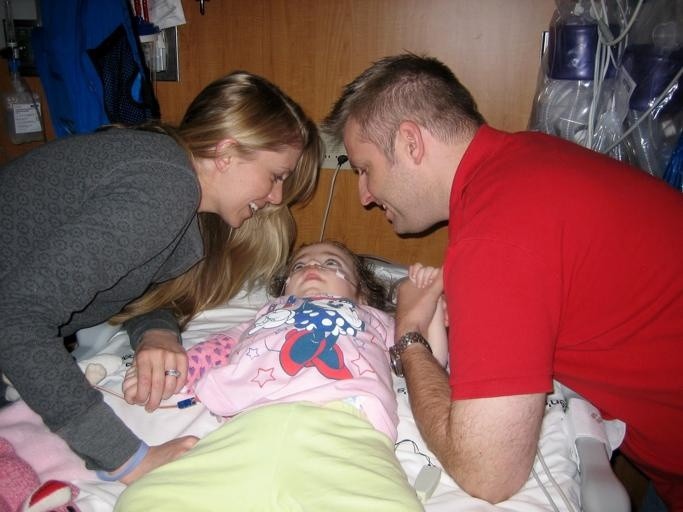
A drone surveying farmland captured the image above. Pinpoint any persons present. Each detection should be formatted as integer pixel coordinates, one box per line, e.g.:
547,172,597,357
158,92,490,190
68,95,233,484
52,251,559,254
317,47,683,510
122,238,449,510
0,69,324,491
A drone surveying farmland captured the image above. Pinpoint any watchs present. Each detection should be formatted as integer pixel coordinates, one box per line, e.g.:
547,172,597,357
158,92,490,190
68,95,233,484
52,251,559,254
388,331,434,378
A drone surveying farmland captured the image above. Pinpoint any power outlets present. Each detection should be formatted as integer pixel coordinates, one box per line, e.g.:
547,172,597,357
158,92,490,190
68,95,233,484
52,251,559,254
319,125,351,170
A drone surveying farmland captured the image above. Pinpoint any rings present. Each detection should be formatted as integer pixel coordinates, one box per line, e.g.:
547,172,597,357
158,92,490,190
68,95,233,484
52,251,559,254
165,370,180,377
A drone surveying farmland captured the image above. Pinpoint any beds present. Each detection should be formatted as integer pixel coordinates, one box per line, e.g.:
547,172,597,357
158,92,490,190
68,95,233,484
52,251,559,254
0,257,634,512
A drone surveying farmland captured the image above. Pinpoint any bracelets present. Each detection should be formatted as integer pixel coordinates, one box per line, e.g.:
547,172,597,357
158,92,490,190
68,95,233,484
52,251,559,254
94,440,150,482
